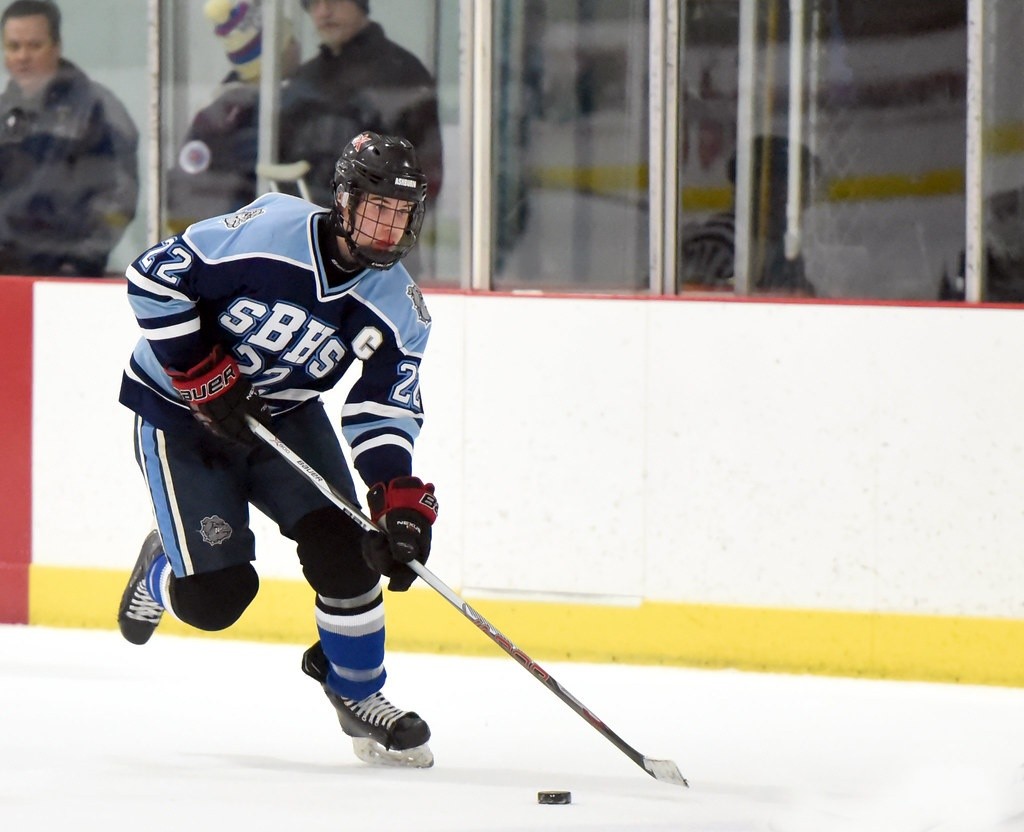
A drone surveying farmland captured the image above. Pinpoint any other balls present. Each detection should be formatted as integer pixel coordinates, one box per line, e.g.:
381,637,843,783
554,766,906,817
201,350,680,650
537,790,572,805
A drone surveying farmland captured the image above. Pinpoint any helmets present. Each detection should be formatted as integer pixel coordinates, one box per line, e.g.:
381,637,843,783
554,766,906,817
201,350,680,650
330,130,430,271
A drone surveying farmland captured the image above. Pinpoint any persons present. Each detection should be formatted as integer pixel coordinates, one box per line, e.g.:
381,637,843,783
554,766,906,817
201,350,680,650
0,1,140,277
939,186,1024,304
119,131,438,768
682,135,820,296
166,0,437,282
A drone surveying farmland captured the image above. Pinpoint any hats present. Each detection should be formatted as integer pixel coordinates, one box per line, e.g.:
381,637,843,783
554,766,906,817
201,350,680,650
204,0,296,80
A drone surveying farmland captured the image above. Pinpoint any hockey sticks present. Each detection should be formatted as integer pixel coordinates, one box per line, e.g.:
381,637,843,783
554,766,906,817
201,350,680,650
246,413,693,789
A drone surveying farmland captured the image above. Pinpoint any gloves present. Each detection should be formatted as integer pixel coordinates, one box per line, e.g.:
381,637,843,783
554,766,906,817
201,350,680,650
361,476,439,592
164,344,282,468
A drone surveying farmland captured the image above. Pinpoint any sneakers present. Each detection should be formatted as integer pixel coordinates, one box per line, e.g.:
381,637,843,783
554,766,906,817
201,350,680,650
119,528,166,645
302,640,434,769
